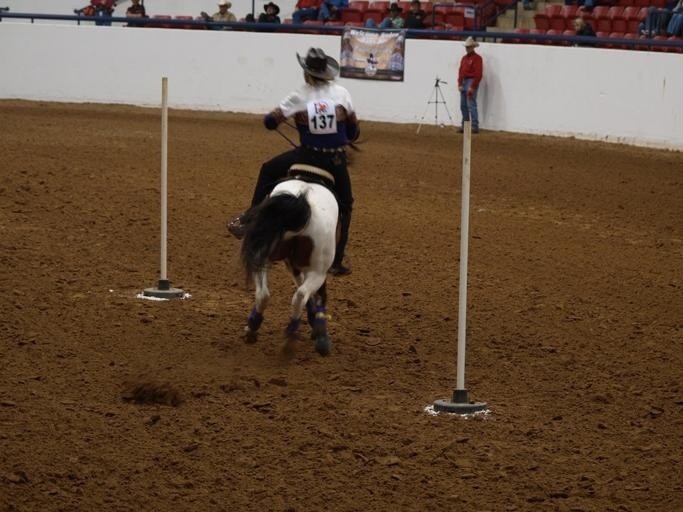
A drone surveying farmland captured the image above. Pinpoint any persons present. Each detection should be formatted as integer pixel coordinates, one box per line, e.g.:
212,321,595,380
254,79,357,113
457,36,482,133
317,0,347,23
225,47,360,276
584,0,593,11
402,1,426,40
665,0,682,37
255,2,280,31
291,0,323,25
638,0,675,38
201,0,237,29
570,19,598,48
366,2,404,37
124,0,144,27
90,0,115,26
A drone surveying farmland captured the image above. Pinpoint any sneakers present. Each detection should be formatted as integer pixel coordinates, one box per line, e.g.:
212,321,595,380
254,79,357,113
230,223,246,236
328,264,352,275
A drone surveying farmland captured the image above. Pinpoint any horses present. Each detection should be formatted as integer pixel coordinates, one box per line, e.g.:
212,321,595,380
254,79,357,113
237,125,374,360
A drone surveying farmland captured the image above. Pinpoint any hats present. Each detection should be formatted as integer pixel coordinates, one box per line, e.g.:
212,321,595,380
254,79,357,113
297,48,339,80
217,1,231,8
264,2,279,14
463,36,479,47
387,3,402,13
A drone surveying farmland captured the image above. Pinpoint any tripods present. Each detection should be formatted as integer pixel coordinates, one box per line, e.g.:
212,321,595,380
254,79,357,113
416,79,460,136
